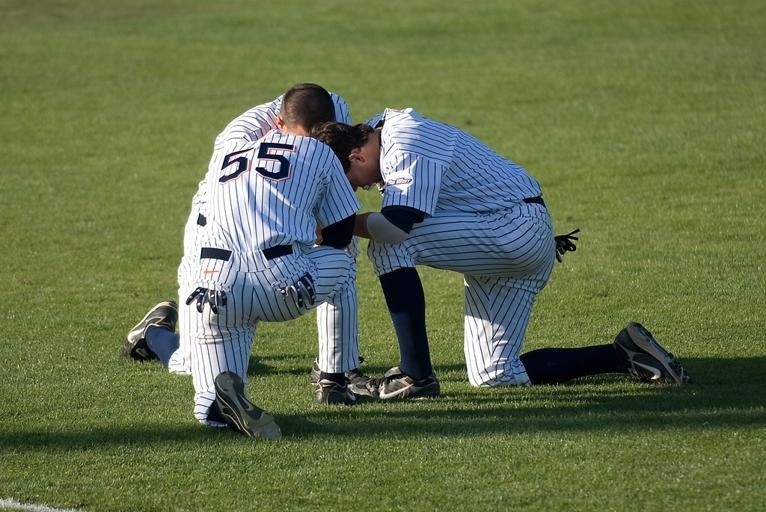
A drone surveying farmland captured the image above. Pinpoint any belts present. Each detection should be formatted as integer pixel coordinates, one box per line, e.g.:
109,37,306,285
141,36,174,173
198,247,295,262
523,197,548,209
196,212,207,226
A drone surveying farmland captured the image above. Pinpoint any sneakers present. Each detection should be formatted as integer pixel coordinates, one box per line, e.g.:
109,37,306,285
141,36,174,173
614,320,693,388
214,370,284,445
309,354,442,406
121,300,179,362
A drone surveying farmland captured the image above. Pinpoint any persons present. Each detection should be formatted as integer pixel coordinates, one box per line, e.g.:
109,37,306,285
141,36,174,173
313,106,691,400
182,128,378,443
118,83,356,376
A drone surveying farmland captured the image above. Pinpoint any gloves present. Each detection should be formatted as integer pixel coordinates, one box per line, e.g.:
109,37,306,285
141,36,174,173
280,273,317,307
553,229,580,263
185,287,228,313
